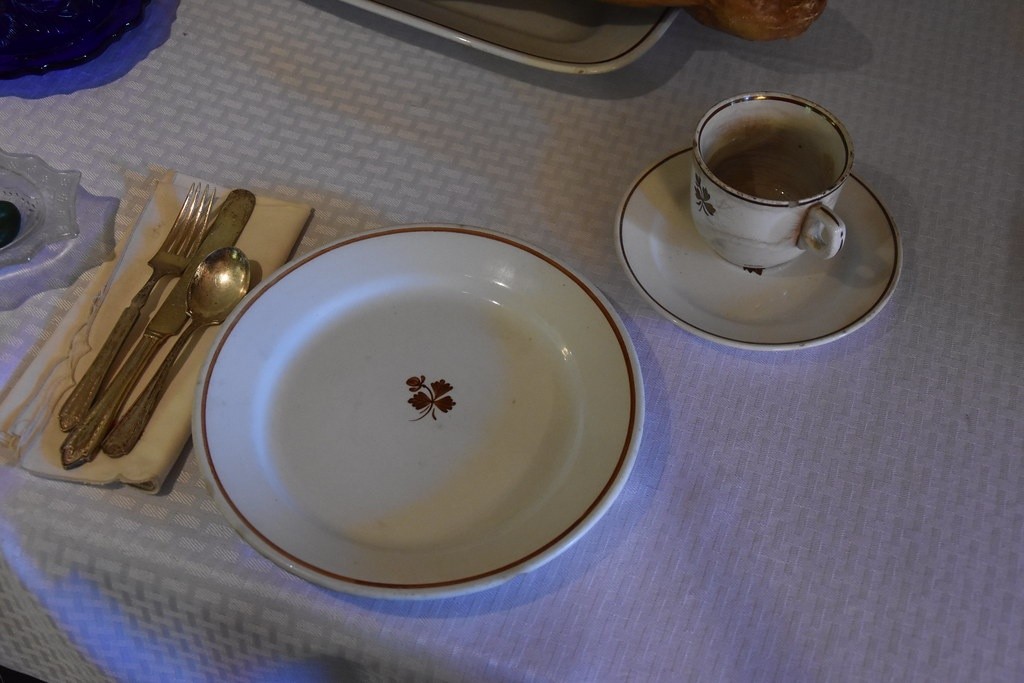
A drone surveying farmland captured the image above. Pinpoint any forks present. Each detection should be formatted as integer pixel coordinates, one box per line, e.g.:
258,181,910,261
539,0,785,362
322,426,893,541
60,182,216,430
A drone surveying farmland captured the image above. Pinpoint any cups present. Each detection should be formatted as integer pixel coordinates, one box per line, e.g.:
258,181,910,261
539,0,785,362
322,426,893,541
693,91,855,270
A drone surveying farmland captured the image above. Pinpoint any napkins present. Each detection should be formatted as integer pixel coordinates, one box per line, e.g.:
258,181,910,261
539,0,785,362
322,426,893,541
0,168,316,496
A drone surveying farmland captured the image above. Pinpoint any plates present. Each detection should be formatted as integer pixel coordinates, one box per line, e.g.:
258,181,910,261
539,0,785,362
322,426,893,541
191,222,645,600
0,148,81,280
342,0,683,76
611,146,904,352
0,0,179,100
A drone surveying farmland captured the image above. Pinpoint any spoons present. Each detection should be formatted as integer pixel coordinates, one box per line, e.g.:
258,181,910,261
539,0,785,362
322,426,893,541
100,246,251,458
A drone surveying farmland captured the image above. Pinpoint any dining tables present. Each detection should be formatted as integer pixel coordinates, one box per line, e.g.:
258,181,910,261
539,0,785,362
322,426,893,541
0,0,1024,683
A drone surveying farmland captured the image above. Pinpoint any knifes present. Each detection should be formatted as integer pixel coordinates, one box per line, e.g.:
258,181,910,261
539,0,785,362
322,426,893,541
61,186,255,467
